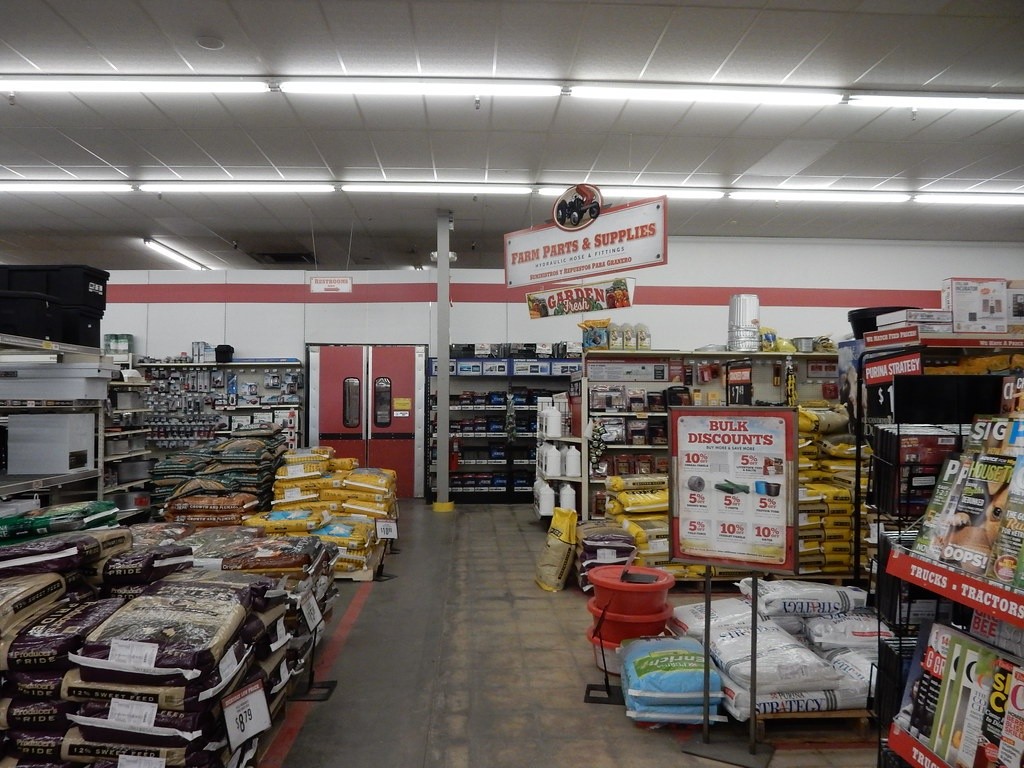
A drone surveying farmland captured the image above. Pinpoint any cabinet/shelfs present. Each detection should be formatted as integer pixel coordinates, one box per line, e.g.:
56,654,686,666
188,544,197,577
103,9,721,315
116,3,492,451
0,332,303,520
422,352,1023,768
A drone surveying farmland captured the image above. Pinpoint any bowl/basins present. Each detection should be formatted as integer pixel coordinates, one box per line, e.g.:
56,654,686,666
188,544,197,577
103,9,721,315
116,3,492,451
587,596,672,644
588,626,672,675
589,565,675,614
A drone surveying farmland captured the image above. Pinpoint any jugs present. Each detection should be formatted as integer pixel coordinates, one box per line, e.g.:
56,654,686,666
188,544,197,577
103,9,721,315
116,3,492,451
288,411,296,449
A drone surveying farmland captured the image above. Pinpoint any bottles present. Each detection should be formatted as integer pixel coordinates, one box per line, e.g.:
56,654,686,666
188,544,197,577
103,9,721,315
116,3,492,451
558,482,575,511
561,445,581,477
533,477,555,516
538,442,561,477
540,407,561,438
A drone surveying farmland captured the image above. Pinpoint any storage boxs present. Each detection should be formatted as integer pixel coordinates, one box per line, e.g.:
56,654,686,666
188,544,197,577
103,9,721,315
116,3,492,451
253,413,272,429
231,415,251,431
0,263,111,348
280,433,301,450
878,277,1023,337
427,342,583,375
214,414,231,432
273,410,301,431
215,344,234,363
